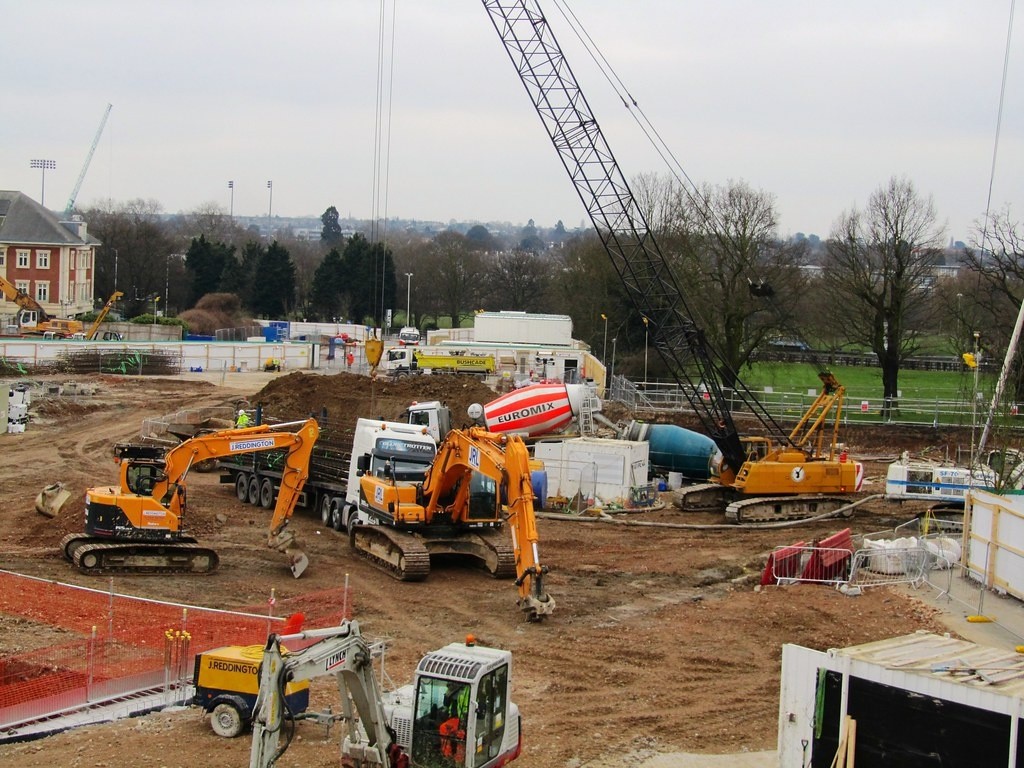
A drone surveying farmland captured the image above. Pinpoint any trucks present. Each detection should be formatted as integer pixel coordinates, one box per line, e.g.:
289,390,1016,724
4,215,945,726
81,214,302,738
776,627,1024,768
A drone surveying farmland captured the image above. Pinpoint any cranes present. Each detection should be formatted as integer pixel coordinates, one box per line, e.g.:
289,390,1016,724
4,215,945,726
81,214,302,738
64,102,114,212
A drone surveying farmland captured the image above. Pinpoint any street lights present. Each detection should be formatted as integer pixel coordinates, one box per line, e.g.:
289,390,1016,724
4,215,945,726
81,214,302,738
267,180,272,242
641,317,648,407
110,247,118,309
404,272,414,328
957,293,962,337
228,180,234,242
600,313,607,366
609,339,617,401
969,331,981,466
30,159,56,206
165,254,178,318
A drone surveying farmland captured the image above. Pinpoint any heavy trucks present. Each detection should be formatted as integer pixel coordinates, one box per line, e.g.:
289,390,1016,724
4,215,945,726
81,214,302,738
216,417,437,535
385,348,498,381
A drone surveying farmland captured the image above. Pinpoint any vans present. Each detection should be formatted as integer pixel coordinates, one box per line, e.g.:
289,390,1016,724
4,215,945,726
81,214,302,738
397,326,422,346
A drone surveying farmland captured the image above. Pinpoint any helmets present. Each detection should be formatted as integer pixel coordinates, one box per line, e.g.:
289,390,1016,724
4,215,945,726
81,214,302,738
350,352,352,354
238,410,244,415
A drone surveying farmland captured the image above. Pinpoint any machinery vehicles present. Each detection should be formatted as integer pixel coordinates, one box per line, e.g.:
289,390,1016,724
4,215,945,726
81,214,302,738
348,427,556,622
60,416,320,579
248,618,523,768
481,0,864,524
883,297,1024,533
0,275,125,342
398,386,601,458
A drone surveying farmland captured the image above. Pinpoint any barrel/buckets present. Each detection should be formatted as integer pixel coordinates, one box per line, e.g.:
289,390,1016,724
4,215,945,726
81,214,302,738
652,478,666,491
668,471,682,490
230,361,248,372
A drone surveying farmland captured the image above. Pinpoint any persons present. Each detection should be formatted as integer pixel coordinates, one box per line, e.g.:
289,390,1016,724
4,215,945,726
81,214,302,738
530,370,533,381
276,359,280,372
347,352,354,370
444,682,470,719
235,410,248,428
321,403,328,427
